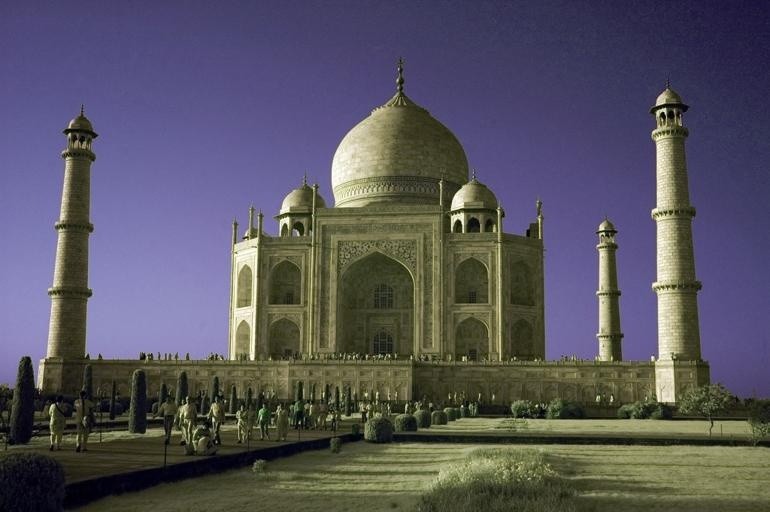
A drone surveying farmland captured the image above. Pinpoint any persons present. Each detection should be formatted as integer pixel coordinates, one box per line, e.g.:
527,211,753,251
97,352,102,360
595,393,615,401
72,390,97,452
650,351,656,362
48,394,71,451
